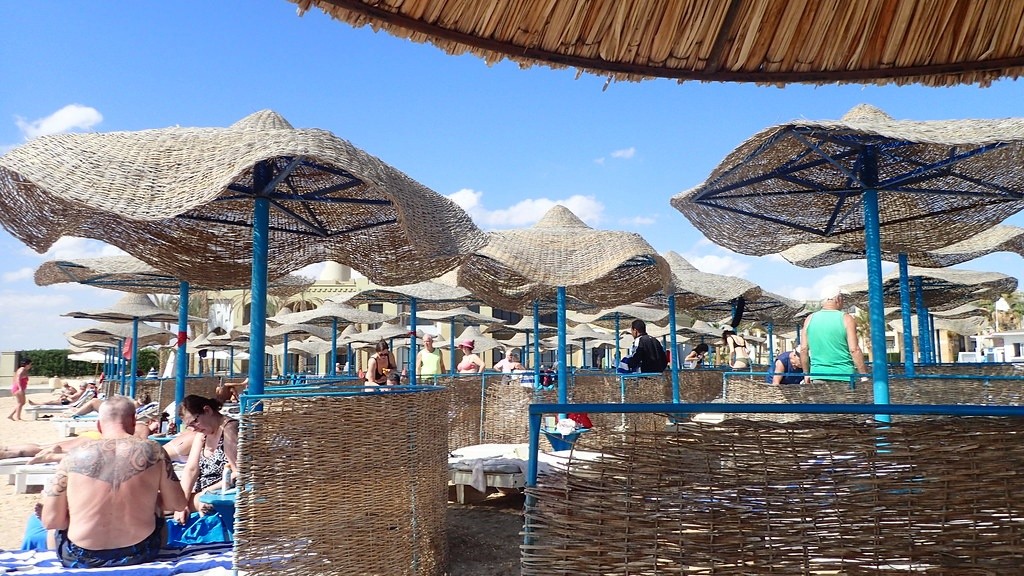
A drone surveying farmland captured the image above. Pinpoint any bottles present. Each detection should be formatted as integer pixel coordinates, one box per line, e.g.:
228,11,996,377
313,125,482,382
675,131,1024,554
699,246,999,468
222,464,231,492
165,418,177,438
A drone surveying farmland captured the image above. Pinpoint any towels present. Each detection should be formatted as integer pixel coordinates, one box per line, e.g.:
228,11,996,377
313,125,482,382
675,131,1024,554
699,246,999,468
16,541,318,576
457,452,503,494
556,419,583,439
615,355,640,374
483,456,552,483
20,511,233,551
511,369,535,387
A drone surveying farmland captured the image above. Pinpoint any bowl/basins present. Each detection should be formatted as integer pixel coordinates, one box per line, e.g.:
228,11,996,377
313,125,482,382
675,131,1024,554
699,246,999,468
539,427,590,452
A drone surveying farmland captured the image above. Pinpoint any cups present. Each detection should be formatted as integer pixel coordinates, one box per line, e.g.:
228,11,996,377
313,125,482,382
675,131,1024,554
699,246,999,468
544,417,555,432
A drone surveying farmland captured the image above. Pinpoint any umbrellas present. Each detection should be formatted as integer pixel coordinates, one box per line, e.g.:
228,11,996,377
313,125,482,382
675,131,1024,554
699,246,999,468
1,105,1024,431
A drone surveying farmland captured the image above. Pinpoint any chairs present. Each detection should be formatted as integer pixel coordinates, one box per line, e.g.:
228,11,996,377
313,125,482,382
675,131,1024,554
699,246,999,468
446,443,617,503
0,388,312,576
692,399,724,424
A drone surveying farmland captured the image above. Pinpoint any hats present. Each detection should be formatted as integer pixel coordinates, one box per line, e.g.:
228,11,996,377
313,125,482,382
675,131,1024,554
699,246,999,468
88,378,96,383
459,339,474,350
821,285,851,302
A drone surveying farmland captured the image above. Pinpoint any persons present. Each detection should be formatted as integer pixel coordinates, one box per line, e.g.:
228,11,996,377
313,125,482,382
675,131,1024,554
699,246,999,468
7,287,870,567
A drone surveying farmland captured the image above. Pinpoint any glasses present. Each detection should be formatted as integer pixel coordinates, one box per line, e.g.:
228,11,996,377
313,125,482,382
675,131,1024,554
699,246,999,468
185,414,200,430
376,352,389,357
401,375,409,379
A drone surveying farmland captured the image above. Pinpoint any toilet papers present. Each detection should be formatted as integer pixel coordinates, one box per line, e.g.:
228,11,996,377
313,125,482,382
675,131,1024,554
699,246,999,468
544,417,555,432
